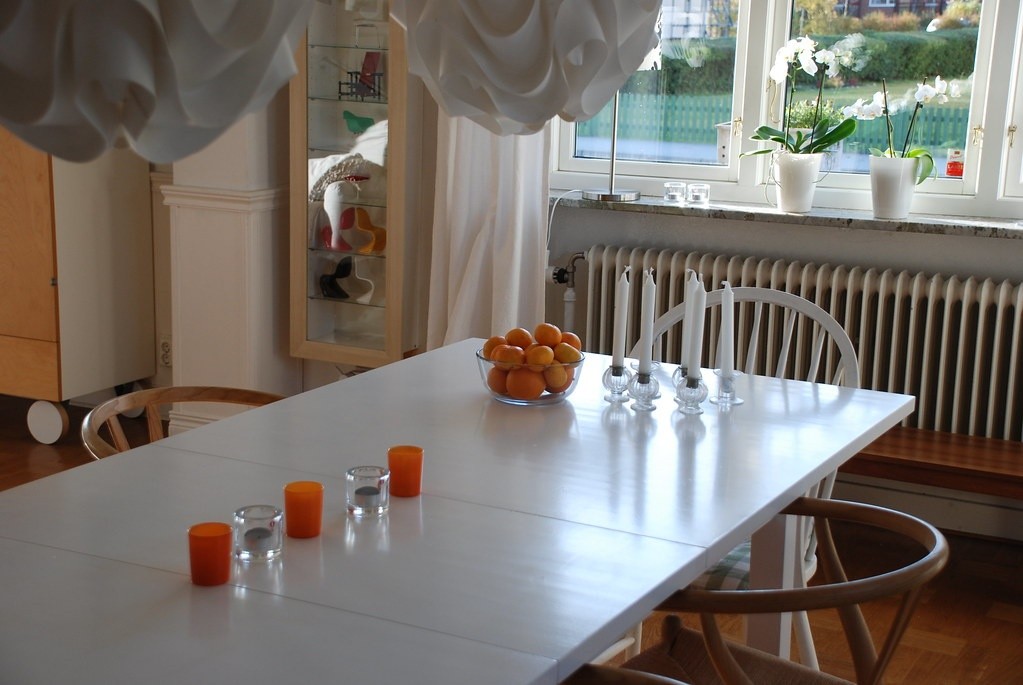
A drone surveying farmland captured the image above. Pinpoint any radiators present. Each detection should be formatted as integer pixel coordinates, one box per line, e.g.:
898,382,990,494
547,243,1023,441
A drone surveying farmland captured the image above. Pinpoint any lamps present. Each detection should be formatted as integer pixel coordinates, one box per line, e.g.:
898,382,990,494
581,5,664,203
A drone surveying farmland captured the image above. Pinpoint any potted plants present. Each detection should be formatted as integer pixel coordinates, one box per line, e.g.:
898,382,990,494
713,98,842,166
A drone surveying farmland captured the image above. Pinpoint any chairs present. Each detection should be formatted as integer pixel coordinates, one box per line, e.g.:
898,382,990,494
560,496,949,685
626,286,860,671
77,385,285,461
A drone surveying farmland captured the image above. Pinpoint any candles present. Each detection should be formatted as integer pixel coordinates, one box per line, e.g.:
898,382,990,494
638,266,657,383
681,268,707,388
612,265,630,376
720,281,735,391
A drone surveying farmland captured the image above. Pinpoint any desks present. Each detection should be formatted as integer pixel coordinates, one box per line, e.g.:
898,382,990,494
0,339,917,685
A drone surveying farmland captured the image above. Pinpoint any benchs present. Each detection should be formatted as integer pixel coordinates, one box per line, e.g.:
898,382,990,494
838,427,1023,501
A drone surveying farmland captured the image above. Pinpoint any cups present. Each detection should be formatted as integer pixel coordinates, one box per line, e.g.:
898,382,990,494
188,523,233,587
688,183,709,203
664,182,686,202
232,504,283,564
284,481,324,538
346,466,391,517
388,446,424,498
946,148,964,176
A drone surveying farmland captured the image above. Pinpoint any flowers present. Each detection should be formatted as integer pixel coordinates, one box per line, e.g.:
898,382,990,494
784,98,843,130
819,31,873,120
840,75,961,185
738,34,857,159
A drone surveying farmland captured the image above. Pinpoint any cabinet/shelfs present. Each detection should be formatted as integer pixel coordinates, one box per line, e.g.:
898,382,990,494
289,0,423,372
0,124,156,444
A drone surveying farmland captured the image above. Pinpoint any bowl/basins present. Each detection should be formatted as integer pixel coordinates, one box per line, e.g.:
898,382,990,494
476,347,586,406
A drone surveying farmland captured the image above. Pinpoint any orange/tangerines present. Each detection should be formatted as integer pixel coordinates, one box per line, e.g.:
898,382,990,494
482,322,582,400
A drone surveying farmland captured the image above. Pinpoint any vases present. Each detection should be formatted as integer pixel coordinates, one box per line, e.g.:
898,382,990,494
869,154,919,219
772,153,823,213
